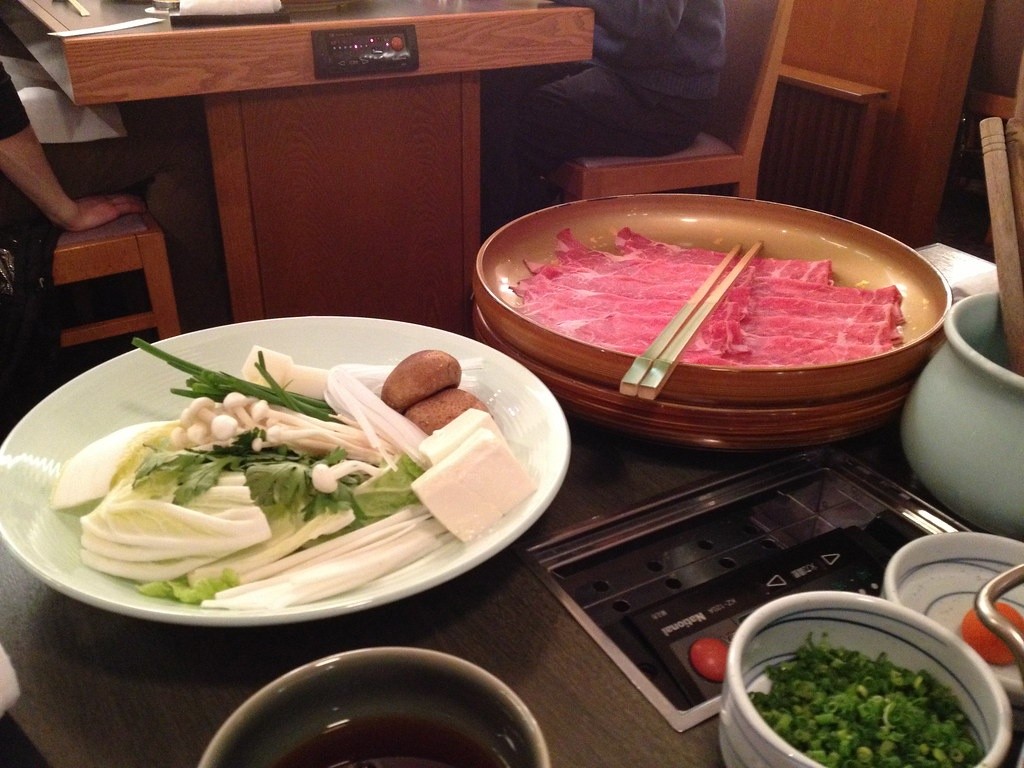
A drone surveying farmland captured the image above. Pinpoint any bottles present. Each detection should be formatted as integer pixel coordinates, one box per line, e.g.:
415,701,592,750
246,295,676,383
899,288,1024,535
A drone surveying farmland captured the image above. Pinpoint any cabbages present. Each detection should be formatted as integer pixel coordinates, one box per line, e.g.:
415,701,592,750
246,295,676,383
45,419,433,605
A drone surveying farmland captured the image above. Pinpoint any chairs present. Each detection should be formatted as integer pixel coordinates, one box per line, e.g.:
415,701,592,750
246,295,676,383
544,0,794,204
52,214,181,347
963,0,1024,251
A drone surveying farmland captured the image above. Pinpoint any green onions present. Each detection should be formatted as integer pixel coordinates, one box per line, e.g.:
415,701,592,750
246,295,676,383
131,337,338,424
746,630,981,768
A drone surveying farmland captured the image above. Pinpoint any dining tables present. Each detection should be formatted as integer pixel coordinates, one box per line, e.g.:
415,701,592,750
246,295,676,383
0,243,1024,768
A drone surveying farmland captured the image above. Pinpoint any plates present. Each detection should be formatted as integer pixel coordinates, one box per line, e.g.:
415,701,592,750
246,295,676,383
0,314,572,628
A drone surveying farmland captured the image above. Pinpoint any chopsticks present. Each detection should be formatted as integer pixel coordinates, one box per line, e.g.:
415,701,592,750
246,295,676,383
979,115,1024,378
68,0,90,17
618,241,764,401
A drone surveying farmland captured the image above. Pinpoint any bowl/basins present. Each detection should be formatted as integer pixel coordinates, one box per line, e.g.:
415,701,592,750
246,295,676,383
717,590,1015,768
471,192,953,453
877,530,1024,743
196,644,551,768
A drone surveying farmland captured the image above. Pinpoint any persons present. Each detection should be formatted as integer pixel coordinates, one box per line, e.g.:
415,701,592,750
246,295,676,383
481,0,726,241
0,62,147,429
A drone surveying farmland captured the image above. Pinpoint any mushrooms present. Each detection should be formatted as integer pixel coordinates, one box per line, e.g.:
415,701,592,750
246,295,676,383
378,349,493,435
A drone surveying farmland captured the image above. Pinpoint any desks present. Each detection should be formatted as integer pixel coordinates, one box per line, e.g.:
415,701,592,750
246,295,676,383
0,0,595,340
